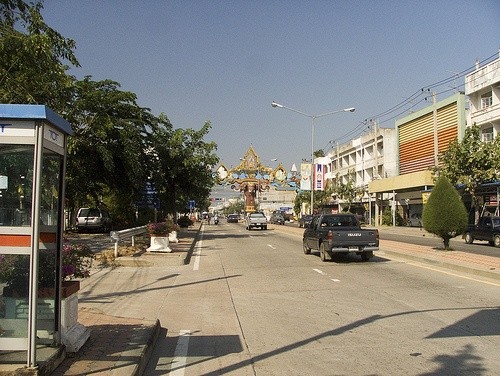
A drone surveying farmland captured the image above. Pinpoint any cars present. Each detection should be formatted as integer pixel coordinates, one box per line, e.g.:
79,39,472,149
202,209,299,226
227,213,238,223
297,214,315,228
246,213,268,230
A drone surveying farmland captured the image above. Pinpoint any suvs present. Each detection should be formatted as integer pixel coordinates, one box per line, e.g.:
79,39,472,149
74,206,108,234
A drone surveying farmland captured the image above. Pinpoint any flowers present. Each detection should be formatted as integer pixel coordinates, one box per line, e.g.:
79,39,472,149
0,241,93,286
145,221,180,237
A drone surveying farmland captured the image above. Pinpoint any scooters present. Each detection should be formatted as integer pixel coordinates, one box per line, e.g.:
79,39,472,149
213,216,220,225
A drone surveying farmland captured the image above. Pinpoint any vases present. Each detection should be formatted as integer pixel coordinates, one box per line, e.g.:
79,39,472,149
3,280,91,353
146,234,172,253
168,231,179,243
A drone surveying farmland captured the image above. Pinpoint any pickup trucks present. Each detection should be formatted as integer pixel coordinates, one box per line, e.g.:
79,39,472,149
302,214,380,262
462,215,500,248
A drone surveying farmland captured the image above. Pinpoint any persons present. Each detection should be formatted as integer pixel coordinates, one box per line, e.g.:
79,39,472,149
214,215,219,222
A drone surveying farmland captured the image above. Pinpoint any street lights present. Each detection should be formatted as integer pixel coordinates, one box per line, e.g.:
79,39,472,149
271,102,356,214
239,157,279,209
